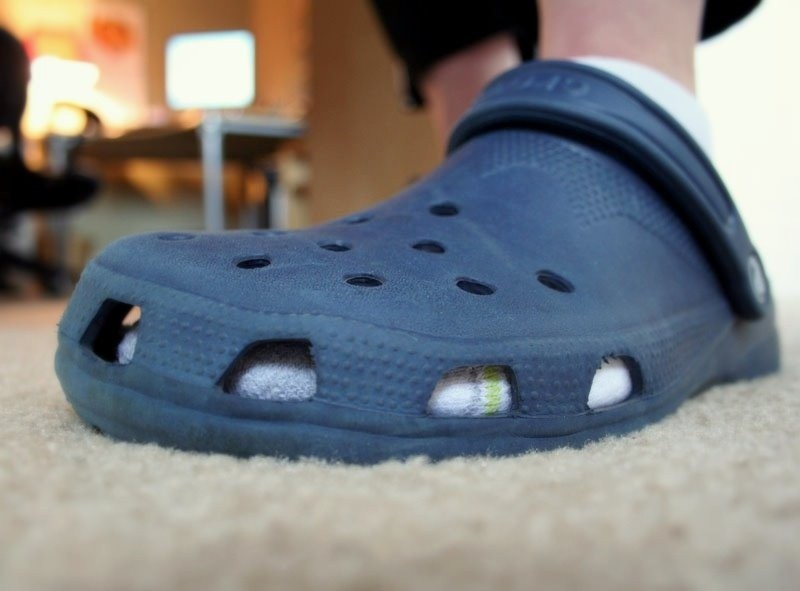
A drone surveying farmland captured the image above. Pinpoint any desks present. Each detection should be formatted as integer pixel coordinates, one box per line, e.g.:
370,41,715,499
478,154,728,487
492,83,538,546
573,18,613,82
39,115,306,295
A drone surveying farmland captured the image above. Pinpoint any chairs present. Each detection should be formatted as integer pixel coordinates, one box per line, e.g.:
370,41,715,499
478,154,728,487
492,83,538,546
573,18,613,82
0,27,105,298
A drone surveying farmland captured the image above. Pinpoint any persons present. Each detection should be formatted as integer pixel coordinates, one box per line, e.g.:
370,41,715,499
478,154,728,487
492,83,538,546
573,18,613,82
55,0,782,465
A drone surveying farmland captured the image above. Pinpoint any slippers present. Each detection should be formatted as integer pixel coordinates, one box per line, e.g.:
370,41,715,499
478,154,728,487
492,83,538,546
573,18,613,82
53,58,779,460
